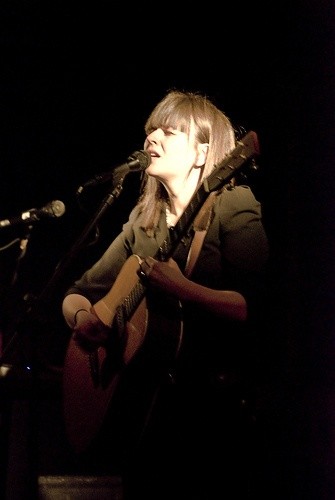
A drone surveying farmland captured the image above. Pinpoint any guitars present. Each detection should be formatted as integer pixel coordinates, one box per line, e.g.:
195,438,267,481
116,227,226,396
65,126,258,431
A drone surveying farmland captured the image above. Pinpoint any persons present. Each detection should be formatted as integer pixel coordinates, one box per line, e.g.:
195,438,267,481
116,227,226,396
61,91,267,500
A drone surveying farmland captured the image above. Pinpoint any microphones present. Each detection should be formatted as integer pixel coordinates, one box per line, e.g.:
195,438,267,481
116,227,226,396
0,200,65,227
76,149,151,195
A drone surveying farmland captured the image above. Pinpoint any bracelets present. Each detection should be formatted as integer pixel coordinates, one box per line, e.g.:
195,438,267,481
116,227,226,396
74,309,89,326
